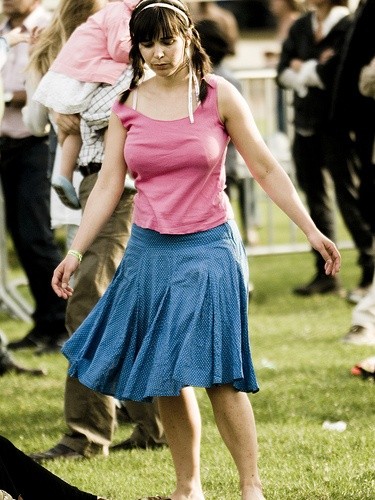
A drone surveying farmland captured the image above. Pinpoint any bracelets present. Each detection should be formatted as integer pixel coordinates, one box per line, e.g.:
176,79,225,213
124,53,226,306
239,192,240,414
66,249,83,262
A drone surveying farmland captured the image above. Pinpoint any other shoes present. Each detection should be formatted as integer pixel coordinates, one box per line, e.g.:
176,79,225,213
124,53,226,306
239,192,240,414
293,277,335,296
52,176,81,210
0,362,44,378
30,444,83,463
6,334,38,349
62,459,62,460
358,253,375,287
111,438,163,449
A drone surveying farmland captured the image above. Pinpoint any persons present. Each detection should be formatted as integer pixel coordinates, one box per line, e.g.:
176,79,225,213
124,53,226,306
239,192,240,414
0,0,375,462
51,0,341,500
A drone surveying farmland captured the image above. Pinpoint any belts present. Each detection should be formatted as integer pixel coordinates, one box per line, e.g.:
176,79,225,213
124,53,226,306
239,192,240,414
79,163,102,177
